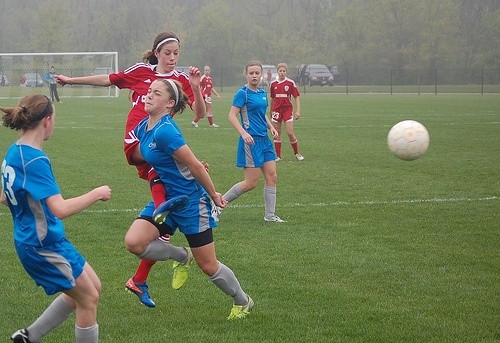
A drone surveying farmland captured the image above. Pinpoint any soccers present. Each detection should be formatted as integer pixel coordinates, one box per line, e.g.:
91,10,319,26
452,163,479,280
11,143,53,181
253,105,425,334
387,119,431,160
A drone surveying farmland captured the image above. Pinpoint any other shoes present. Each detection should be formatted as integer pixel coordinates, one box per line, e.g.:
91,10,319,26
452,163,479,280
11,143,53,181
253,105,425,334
192,120,199,128
294,153,304,161
275,156,283,161
59,101,62,104
208,123,220,128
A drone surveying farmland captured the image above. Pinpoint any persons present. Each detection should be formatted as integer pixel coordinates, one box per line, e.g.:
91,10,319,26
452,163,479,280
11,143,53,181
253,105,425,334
192,66,220,128
211,61,288,223
0,95,111,343
48,66,62,104
269,63,304,162
267,70,272,90
53,33,206,307
125,78,255,320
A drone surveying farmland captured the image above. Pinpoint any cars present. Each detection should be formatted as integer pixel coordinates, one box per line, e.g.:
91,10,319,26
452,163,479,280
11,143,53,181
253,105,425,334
258,65,278,87
298,64,340,86
24,73,45,87
0,75,9,86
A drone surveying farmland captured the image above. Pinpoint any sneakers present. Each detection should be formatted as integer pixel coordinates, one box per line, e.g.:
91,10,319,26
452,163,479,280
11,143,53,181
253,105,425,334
153,195,190,225
125,276,156,308
226,294,255,320
171,246,194,290
263,215,288,223
211,200,223,222
11,328,40,343
